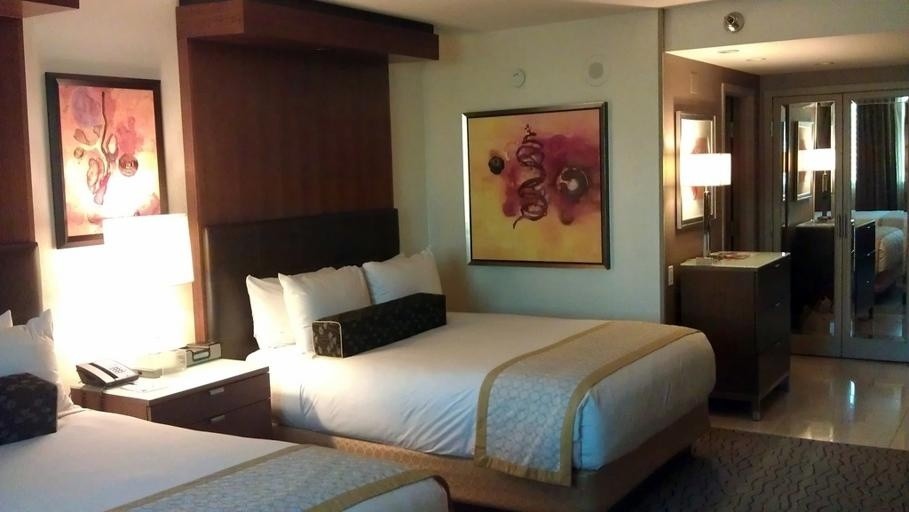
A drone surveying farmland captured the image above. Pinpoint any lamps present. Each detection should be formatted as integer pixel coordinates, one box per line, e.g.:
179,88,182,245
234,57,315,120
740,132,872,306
798,146,838,220
100,212,194,379
680,152,735,264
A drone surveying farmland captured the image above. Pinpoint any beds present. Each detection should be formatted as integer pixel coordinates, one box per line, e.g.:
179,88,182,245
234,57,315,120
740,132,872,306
0,241,454,512
812,210,908,306
202,208,716,511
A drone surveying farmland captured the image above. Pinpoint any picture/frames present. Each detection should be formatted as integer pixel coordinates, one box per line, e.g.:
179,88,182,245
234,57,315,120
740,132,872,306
792,120,818,201
43,69,172,249
675,111,723,231
459,103,610,272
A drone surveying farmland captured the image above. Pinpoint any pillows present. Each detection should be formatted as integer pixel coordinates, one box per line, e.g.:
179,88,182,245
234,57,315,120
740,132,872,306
249,247,451,358
0,305,74,443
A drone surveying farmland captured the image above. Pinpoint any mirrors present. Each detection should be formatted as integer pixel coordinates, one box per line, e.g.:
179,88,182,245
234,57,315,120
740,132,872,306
774,94,837,335
846,92,909,340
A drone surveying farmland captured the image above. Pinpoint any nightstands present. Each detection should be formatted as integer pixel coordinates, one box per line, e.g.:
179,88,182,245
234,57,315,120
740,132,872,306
67,360,274,438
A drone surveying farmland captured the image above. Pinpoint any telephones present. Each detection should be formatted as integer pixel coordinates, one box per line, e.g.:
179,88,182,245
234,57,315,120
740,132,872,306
76,360,139,385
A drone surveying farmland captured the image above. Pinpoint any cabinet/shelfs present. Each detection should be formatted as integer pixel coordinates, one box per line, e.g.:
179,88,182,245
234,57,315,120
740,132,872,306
680,251,795,422
796,212,876,323
